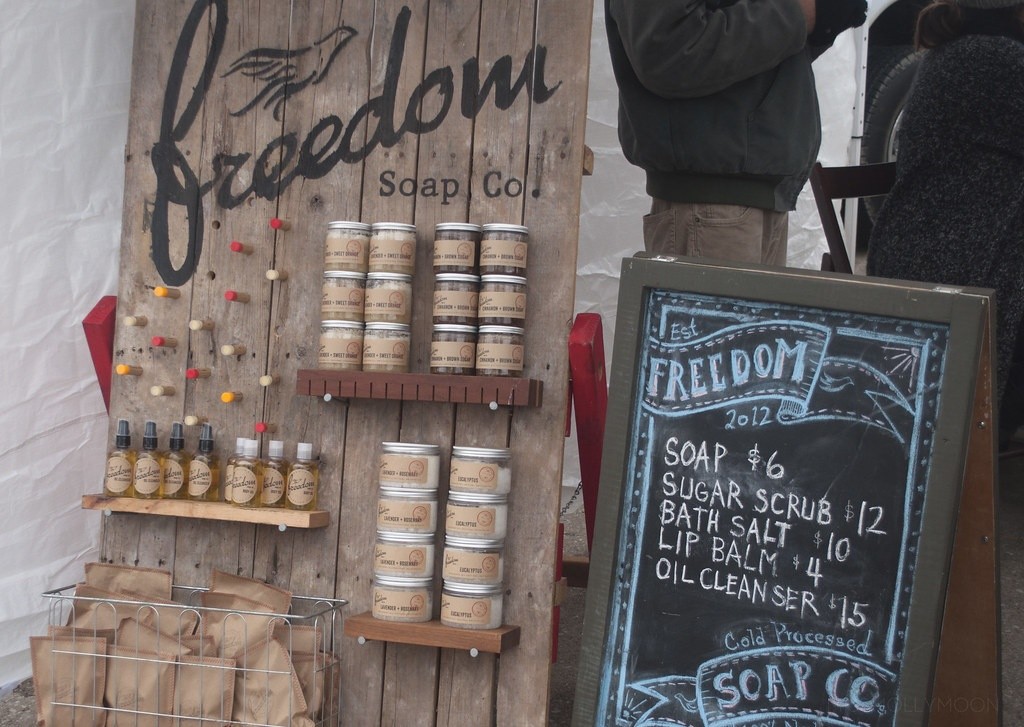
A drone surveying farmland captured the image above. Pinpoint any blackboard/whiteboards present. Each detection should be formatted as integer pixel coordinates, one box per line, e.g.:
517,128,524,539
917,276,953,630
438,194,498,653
570,251,1002,727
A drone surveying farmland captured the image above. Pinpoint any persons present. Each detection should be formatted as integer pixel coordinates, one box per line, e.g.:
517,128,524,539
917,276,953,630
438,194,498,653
867,0,1024,458
605,0,869,266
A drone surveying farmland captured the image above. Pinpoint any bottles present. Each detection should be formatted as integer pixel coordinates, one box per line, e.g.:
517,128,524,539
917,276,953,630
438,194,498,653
224,437,250,502
134,420,163,500
370,440,511,628
188,422,220,501
317,221,416,374
262,440,290,509
103,418,135,498
161,422,191,500
231,440,264,508
284,442,317,511
432,221,530,375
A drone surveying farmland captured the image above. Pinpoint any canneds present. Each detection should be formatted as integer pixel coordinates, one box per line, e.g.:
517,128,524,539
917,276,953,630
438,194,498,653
318,221,416,373
429,222,528,376
441,445,513,630
372,442,442,623
